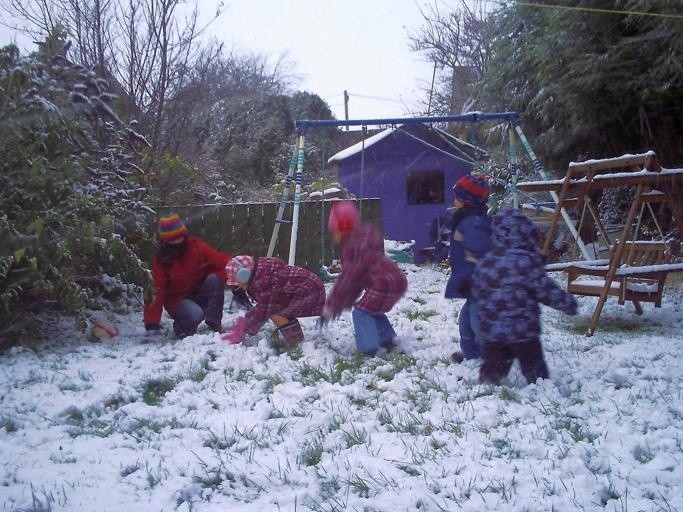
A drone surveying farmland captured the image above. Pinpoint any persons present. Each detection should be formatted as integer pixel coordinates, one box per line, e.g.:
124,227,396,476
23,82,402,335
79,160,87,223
465,208,578,384
316,195,408,357
220,255,325,356
444,172,494,367
143,209,232,339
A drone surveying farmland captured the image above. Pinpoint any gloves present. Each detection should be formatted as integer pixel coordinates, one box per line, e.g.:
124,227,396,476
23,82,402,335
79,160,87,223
146,323,160,331
222,317,250,344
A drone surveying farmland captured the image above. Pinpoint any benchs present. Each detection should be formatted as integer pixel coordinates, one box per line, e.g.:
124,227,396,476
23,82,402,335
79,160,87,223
564,239,674,307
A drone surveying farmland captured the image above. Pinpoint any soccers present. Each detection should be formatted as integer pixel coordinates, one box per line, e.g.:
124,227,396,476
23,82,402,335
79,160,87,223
92,322,117,342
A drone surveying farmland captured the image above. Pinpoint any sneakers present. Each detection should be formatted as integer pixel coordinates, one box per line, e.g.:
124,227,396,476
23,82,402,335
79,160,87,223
452,353,464,363
206,320,223,333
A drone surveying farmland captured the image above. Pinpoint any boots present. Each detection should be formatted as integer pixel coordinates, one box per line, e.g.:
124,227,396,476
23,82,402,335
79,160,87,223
278,319,303,344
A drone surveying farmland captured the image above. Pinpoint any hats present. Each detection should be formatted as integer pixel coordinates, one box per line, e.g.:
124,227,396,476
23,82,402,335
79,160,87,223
327,203,358,232
225,256,254,286
159,213,187,242
453,174,489,206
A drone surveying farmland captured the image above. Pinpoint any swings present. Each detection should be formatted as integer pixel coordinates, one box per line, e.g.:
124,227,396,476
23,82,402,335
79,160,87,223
388,122,577,251
567,189,676,307
318,124,367,281
